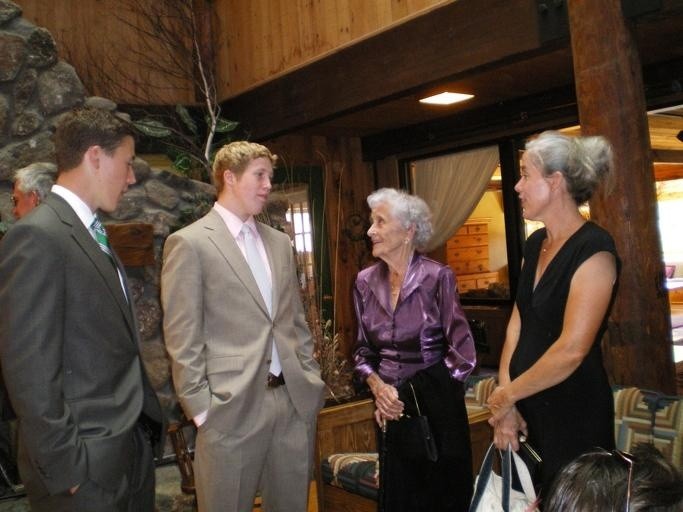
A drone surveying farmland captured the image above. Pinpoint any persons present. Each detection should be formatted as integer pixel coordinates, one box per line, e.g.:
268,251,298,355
534,443,681,512
352,187,476,512
487,132,623,512
7,157,62,224
0,103,168,512
162,138,326,511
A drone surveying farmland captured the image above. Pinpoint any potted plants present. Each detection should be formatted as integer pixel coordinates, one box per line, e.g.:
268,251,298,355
305,318,355,407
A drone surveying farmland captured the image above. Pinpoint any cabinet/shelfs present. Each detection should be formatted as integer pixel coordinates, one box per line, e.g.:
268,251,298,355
431,221,499,296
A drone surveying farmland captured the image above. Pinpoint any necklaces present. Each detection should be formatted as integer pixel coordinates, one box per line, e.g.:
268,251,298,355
390,281,397,296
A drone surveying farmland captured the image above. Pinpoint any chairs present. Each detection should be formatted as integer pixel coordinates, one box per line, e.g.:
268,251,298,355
312,375,497,512
609,384,683,479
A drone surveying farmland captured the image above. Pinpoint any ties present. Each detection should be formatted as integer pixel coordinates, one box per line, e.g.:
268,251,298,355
92,216,116,269
241,225,282,378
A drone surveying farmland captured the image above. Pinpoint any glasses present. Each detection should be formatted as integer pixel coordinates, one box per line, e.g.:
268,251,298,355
579,448,637,511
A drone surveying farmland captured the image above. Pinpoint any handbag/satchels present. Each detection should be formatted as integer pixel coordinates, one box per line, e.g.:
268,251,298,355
469,441,540,512
380,380,438,471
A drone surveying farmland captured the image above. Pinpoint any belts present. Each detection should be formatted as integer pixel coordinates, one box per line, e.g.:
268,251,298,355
265,373,285,389
